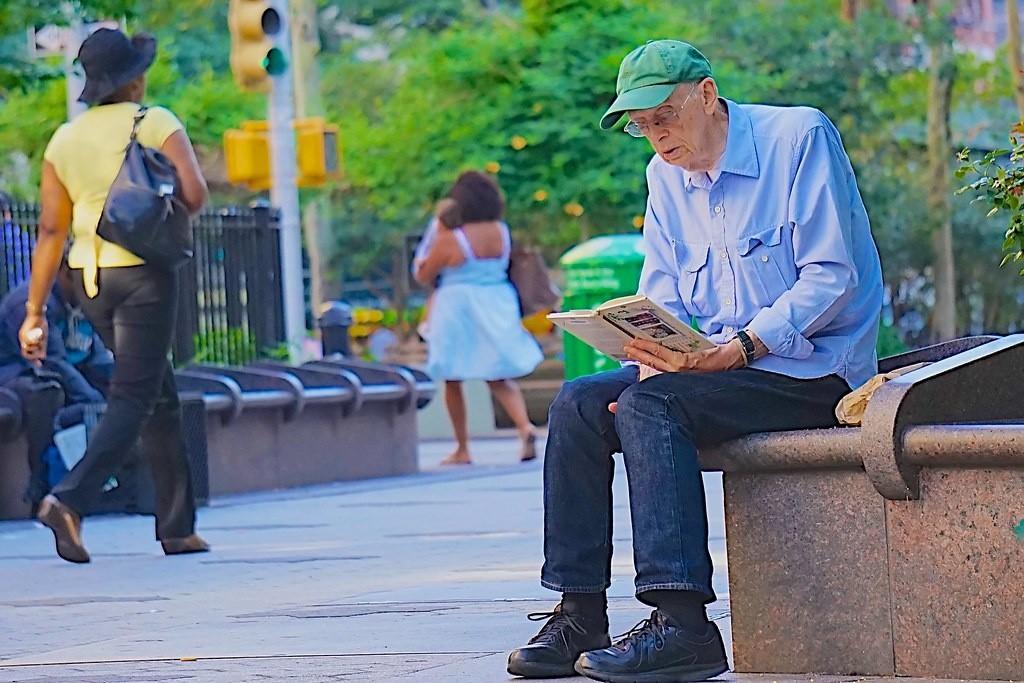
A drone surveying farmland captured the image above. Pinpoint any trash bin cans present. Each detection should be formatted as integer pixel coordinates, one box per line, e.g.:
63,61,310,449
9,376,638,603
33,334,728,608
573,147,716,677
558,232,651,382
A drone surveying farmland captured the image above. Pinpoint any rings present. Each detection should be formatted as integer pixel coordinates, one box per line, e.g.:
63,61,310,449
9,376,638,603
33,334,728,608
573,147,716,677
21,343,26,348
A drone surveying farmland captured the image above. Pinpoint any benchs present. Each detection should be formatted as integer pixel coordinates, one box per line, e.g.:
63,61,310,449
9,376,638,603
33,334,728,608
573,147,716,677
0,358,439,521
699,333,1024,683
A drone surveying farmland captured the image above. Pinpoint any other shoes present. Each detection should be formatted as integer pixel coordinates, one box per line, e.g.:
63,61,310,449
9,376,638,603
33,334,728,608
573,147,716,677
519,425,536,460
440,454,471,465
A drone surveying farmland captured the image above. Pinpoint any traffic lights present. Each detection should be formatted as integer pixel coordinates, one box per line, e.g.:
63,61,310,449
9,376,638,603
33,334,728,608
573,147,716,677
295,113,342,187
226,2,286,90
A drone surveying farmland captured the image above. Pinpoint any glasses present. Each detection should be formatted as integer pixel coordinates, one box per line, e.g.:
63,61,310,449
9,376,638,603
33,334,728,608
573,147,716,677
624,85,696,138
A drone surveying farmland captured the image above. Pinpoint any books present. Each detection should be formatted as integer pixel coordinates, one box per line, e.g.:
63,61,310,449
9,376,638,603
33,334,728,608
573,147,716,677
544,295,717,362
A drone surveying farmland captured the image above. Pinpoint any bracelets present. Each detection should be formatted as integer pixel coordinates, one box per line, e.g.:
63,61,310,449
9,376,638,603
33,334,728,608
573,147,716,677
26,301,47,313
732,331,757,368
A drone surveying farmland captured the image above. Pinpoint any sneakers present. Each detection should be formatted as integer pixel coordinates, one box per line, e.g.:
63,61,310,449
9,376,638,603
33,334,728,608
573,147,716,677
572,610,730,683
505,603,612,675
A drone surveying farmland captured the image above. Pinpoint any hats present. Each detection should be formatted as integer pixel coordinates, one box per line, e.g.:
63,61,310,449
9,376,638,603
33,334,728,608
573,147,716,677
74,29,158,106
600,39,712,131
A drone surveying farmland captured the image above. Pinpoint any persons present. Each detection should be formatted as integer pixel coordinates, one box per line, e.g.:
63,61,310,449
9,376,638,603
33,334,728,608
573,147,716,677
18,28,211,564
0,242,155,522
507,39,885,683
412,171,542,464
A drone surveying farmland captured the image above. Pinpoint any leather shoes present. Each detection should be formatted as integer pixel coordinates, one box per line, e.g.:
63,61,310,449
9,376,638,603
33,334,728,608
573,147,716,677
157,531,209,553
40,493,91,565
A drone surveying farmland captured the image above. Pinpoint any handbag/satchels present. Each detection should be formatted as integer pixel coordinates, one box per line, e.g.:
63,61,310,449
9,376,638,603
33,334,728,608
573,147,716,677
95,105,194,270
509,248,562,320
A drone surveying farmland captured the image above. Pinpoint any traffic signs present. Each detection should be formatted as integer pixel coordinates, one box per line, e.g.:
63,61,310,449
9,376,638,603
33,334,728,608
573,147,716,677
32,18,124,60
